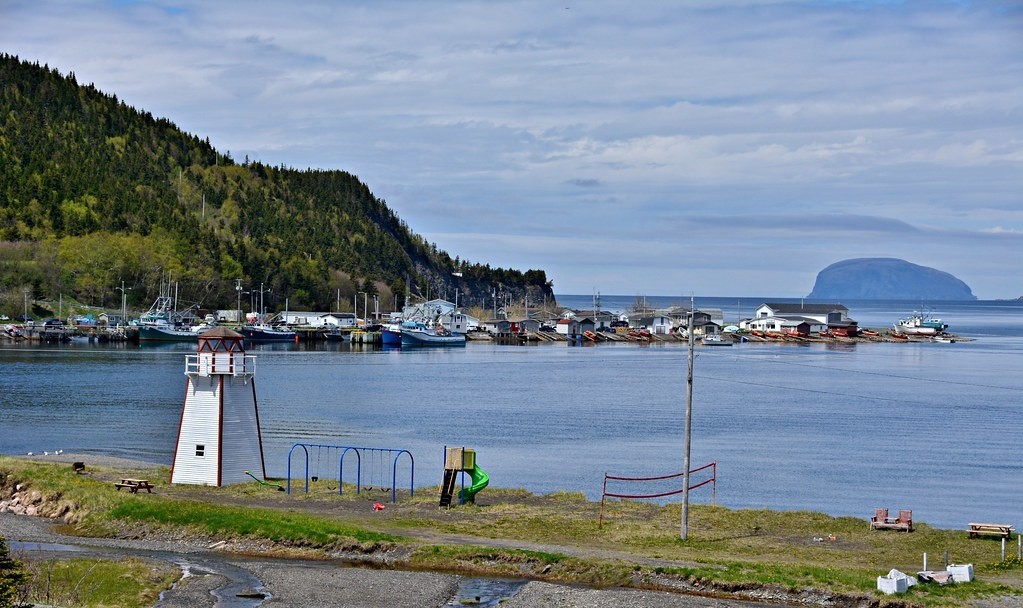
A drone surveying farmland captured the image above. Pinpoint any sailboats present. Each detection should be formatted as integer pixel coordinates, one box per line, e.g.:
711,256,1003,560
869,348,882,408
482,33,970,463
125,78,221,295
323,288,344,340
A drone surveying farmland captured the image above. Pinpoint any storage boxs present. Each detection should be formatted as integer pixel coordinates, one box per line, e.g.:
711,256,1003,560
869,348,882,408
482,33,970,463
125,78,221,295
946,564,973,583
876,576,908,595
918,571,951,585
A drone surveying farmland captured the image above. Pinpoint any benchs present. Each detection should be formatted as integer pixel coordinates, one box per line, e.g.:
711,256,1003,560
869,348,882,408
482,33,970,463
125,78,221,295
972,527,1016,531
966,530,1008,540
115,484,137,492
137,483,154,492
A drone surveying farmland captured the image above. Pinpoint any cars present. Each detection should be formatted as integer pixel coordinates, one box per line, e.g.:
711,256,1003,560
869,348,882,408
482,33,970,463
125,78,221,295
539,325,556,333
615,321,629,327
596,326,615,333
317,322,337,330
12,314,67,327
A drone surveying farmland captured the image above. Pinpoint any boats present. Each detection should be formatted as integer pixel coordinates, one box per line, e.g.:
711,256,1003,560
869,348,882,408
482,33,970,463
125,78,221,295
751,329,764,337
629,329,650,338
679,326,690,339
138,270,198,341
241,321,296,341
701,331,733,346
785,329,805,337
766,330,785,337
862,330,879,336
892,331,908,339
893,303,949,336
585,330,596,339
818,330,847,338
350,273,466,345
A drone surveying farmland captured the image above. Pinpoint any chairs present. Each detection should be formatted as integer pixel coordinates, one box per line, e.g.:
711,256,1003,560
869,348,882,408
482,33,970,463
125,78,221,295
870,508,913,532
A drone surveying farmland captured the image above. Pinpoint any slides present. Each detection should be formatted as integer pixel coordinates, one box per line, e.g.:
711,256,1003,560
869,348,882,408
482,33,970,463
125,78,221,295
458,451,489,505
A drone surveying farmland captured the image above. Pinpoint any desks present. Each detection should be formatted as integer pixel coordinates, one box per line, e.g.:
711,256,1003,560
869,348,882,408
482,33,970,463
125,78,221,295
122,479,152,493
968,523,1013,540
